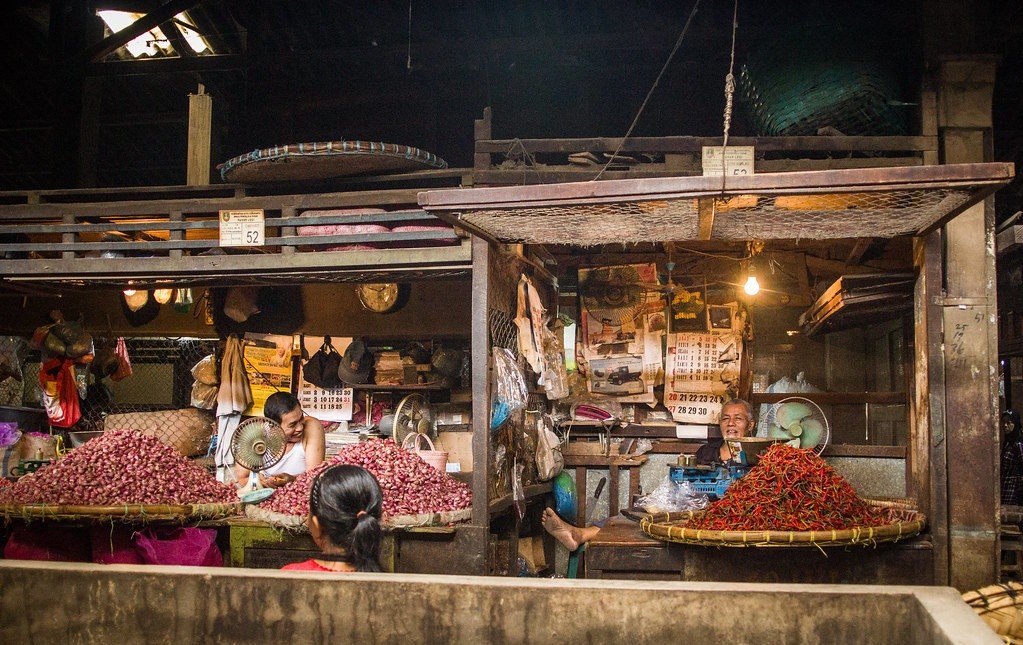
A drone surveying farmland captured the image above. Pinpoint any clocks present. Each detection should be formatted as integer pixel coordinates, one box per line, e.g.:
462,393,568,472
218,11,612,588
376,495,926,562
355,282,399,312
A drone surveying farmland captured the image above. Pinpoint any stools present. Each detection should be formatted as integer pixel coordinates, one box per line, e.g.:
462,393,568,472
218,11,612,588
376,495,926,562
566,543,585,578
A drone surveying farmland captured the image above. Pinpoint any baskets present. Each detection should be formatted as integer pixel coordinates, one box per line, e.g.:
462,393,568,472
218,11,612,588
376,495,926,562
400,433,448,475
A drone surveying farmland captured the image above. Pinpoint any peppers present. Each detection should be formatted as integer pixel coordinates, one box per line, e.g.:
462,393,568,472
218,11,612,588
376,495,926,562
675,438,891,532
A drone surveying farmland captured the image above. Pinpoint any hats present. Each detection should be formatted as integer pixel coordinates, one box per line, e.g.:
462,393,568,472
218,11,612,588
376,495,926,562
337,340,371,385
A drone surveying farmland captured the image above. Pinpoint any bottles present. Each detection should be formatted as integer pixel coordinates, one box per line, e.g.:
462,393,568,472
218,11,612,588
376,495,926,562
677,452,687,467
688,455,697,466
36,447,43,460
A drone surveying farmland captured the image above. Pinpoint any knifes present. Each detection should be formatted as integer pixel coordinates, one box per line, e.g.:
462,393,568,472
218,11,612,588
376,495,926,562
585,477,607,523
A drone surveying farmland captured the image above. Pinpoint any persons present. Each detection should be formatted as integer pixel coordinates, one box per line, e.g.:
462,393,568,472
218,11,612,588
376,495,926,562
999,409,1023,506
230,392,326,489
281,464,385,572
542,399,756,552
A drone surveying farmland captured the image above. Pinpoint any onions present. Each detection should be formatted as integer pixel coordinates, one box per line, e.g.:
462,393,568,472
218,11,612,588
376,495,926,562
256,435,472,517
0,427,240,505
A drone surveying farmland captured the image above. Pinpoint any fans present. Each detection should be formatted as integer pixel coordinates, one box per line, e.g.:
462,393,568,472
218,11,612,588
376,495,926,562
762,397,830,459
579,259,648,327
230,417,287,496
380,392,434,450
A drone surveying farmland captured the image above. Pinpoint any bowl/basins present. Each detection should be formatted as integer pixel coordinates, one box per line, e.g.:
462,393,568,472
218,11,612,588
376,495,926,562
70,430,101,447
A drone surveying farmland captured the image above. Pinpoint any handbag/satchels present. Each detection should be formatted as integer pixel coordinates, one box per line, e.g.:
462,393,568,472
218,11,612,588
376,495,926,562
40,361,81,428
494,346,528,412
110,338,133,382
538,421,564,482
303,337,343,389
